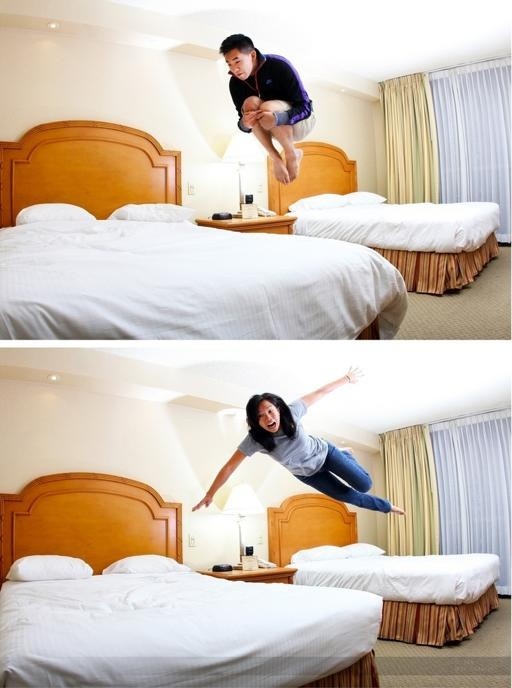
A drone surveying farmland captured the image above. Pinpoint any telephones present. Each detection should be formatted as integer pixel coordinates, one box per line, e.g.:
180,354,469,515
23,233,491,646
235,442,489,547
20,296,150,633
257,559,277,568
257,207,277,217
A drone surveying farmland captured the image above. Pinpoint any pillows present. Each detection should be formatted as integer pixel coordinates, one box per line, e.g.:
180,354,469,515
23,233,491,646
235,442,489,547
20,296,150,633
341,542,386,558
287,193,350,209
343,191,388,206
105,202,198,223
290,545,351,561
101,554,192,575
5,554,94,581
15,202,97,227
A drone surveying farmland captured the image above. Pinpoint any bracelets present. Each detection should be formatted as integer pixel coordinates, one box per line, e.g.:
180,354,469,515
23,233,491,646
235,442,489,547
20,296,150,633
344,374,351,383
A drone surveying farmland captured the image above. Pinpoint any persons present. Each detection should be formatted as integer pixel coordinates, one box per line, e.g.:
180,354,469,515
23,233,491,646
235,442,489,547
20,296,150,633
191,359,406,519
215,33,317,186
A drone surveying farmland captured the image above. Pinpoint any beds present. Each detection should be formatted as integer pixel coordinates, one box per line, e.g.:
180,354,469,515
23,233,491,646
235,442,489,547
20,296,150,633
0,472,376,688
0,120,380,339
267,492,502,648
266,140,501,295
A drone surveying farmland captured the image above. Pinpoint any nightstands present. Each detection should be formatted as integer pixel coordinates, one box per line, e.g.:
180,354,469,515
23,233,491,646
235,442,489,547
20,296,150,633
194,212,298,235
193,562,298,585
